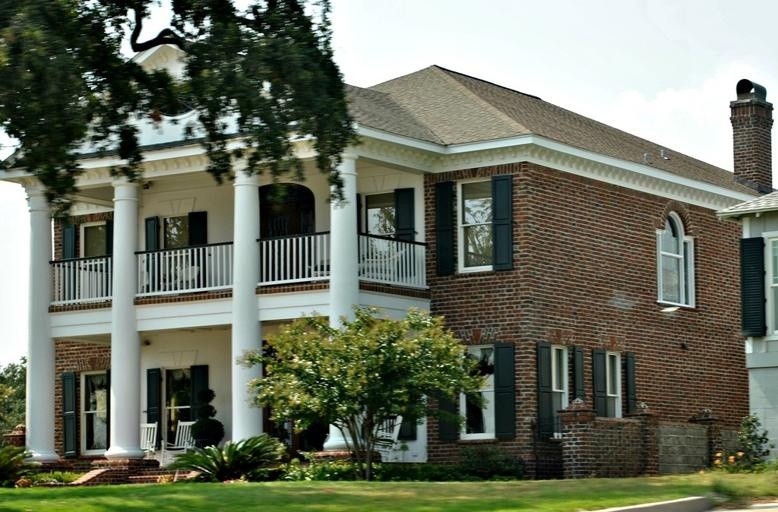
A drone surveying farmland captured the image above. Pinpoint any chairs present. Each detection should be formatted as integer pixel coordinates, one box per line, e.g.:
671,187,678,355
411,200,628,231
160,419,197,467
140,421,158,459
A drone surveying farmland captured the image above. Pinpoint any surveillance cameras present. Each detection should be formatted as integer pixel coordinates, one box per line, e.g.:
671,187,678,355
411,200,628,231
144,336,151,346
143,181,151,189
